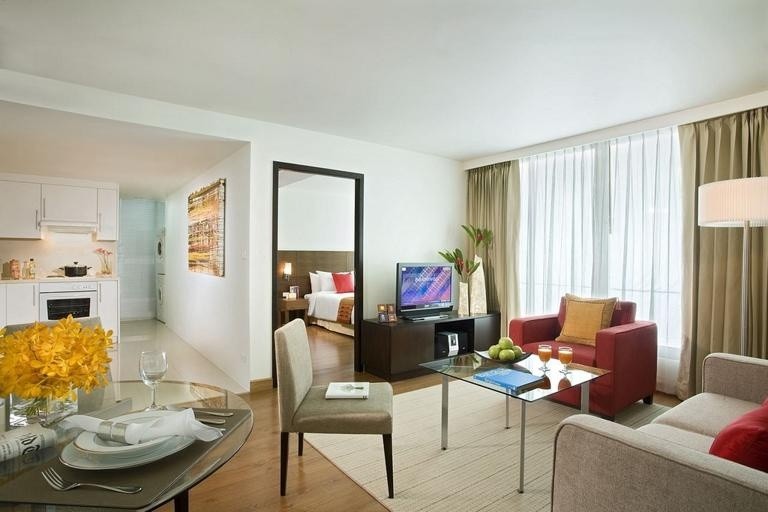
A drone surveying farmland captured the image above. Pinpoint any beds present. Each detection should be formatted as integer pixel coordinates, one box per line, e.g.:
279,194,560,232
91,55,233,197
304,293,354,338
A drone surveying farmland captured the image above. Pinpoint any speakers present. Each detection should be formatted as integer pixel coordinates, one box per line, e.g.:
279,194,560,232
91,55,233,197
438,332,459,357
457,332,469,355
436,333,449,359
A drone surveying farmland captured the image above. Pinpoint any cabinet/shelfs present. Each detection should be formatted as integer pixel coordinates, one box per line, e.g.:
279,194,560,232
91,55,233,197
363,311,501,382
0,172,120,402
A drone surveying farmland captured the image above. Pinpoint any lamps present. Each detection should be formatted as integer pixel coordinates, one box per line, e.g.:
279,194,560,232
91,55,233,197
697,175,767,356
282,263,292,281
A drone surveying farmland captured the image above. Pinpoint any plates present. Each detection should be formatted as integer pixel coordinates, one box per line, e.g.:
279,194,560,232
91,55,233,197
58,434,197,472
74,410,179,459
474,350,533,365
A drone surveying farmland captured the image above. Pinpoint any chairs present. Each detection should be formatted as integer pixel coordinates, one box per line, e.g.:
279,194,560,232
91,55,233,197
274,318,395,499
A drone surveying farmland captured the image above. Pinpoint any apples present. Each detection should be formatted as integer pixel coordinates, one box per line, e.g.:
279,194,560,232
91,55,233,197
499,349,514,362
512,346,522,358
499,337,513,349
488,345,502,358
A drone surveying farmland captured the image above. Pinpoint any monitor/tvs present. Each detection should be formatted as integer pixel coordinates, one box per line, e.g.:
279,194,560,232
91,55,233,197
395,261,456,323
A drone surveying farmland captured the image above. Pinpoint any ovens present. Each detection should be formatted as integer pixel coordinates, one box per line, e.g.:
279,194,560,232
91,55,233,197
39,283,98,321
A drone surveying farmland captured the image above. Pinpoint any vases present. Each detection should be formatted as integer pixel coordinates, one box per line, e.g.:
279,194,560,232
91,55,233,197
8,401,78,427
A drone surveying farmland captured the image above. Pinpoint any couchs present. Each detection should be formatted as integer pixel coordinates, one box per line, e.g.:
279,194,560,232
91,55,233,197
549,350,768,512
510,296,659,421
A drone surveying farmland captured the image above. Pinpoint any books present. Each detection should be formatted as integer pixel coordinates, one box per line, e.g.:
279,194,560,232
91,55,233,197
473,367,544,392
324,381,370,399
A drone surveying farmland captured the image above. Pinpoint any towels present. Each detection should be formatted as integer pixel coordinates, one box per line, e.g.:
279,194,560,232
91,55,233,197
56,408,224,442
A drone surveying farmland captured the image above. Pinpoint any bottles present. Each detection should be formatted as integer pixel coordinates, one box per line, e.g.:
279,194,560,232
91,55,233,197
22,261,29,279
29,258,35,279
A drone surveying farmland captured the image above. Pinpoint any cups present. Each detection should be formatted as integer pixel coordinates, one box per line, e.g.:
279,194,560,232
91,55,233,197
559,347,572,375
139,349,168,412
539,345,551,372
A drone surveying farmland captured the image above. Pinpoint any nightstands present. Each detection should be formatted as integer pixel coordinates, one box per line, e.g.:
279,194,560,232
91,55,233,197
278,298,310,328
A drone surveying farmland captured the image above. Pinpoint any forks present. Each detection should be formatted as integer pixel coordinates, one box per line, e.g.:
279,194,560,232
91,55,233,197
41,467,143,498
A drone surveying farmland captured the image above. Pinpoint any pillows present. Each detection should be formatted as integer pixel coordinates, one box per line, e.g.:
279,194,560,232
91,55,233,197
332,273,354,293
316,271,331,291
709,393,768,473
555,292,618,351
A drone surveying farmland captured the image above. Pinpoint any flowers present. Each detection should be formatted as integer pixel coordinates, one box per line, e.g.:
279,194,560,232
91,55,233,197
95,247,115,274
0,314,114,401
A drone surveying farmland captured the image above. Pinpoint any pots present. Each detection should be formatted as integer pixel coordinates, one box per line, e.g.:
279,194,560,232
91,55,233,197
59,261,93,277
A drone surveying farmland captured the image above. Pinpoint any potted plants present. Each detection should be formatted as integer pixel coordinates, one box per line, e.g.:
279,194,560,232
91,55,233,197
438,249,479,316
461,223,493,314
